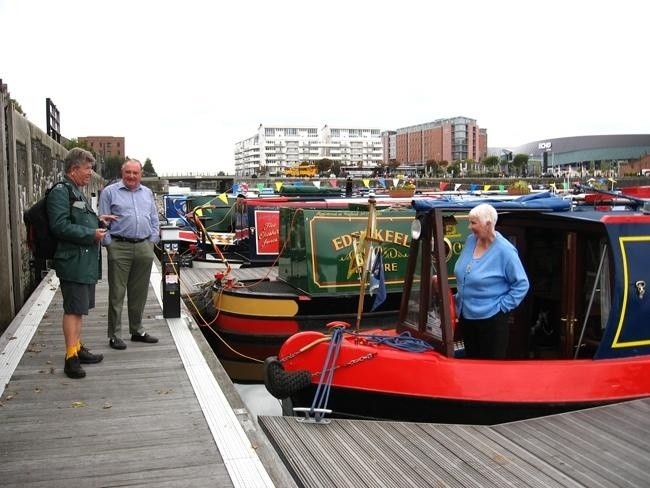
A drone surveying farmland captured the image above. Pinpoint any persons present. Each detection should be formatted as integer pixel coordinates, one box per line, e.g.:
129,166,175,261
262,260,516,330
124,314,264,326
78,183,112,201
44,148,119,379
451,202,530,358
97,157,160,349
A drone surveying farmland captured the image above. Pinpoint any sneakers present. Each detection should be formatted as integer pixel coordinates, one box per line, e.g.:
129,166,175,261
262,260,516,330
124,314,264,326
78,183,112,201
109,337,126,348
78,346,105,363
133,331,158,344
64,353,84,378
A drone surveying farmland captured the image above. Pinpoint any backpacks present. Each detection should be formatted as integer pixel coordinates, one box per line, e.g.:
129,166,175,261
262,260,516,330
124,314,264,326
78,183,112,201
23,180,76,261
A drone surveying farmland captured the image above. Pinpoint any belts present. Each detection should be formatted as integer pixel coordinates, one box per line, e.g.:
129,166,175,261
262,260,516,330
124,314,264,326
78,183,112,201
110,233,149,244
